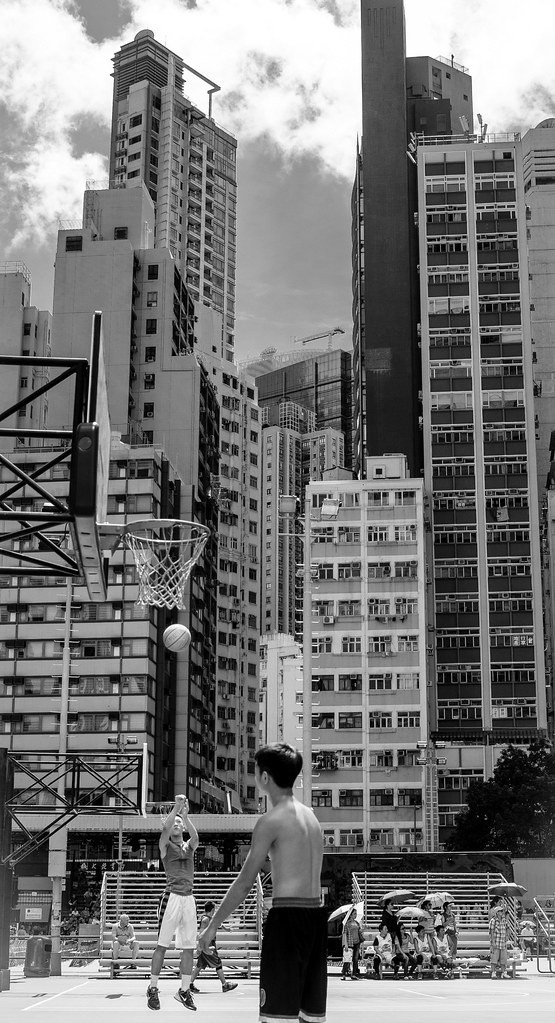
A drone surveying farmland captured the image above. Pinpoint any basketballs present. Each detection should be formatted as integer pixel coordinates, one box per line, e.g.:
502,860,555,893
162,624,192,653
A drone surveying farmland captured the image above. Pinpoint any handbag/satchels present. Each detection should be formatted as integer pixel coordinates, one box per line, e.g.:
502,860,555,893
342,947,353,963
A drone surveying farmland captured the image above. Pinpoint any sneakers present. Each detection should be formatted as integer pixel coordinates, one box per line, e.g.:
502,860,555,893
174,988,196,1011
222,982,238,993
146,985,160,1010
190,983,198,993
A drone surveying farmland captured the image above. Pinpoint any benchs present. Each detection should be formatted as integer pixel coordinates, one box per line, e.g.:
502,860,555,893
101,941,260,978
360,931,529,979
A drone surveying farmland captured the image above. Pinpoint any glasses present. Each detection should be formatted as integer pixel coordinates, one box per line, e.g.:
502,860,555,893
391,903,394,906
400,927,406,930
428,904,433,906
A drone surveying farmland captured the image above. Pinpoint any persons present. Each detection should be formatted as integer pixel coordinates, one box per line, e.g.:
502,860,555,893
373,924,399,979
452,901,488,931
472,876,485,899
534,901,543,918
193,859,242,877
341,909,361,981
440,901,458,960
112,914,139,976
520,922,535,955
28,861,156,935
413,925,440,980
197,743,329,1023
488,896,503,920
190,900,238,993
17,925,28,940
145,795,197,1010
418,900,436,938
432,924,456,980
394,922,417,980
382,898,397,970
545,900,552,919
489,905,512,979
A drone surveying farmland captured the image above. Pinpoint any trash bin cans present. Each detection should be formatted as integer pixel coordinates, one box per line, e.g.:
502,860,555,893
22,936,53,978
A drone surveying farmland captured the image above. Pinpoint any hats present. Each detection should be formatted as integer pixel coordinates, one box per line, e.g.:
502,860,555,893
494,906,503,912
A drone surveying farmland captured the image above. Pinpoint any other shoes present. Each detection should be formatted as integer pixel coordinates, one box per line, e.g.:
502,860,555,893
341,975,358,980
492,971,511,979
131,963,137,969
417,974,439,980
375,974,400,980
114,964,120,974
441,967,451,980
403,975,414,980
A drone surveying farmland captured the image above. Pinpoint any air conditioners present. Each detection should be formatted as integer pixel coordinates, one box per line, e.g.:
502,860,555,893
128,257,157,419
312,523,424,853
197,377,259,805
420,151,541,811
